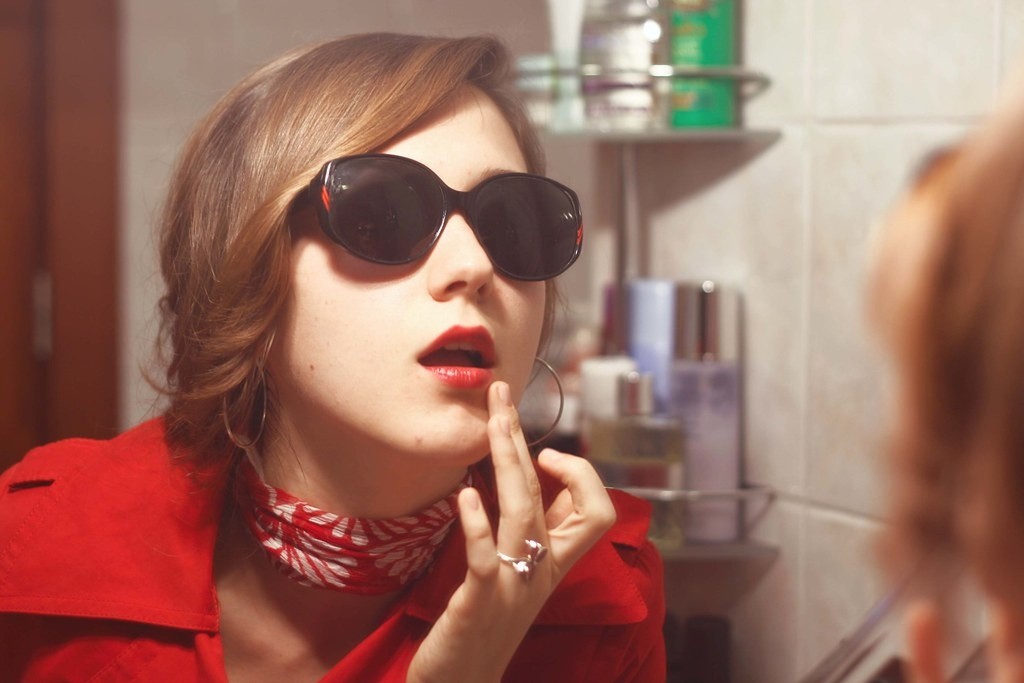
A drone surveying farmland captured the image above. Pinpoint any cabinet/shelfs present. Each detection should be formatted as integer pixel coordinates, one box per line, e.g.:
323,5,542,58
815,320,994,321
511,60,778,565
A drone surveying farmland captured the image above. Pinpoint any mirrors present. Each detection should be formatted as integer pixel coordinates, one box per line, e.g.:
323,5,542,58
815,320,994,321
1,0,1024,683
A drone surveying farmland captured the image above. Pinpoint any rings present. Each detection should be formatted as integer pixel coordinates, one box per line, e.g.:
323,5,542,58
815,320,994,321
495,539,547,581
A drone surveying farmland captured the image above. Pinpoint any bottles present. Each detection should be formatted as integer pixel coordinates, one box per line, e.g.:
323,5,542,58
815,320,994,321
582,371,686,555
671,281,740,537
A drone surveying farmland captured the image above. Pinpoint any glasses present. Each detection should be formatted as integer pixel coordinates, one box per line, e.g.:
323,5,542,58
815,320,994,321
285,153,584,279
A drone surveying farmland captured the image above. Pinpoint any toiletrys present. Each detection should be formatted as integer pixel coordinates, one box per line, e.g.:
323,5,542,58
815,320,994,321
521,278,741,550
512,0,738,132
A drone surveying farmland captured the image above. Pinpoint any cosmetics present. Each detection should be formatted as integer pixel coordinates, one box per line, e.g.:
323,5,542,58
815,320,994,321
506,2,738,127
550,277,744,547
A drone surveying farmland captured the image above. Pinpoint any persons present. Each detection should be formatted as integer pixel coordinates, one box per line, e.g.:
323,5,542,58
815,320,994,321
0,33,666,683
873,95,1024,683
